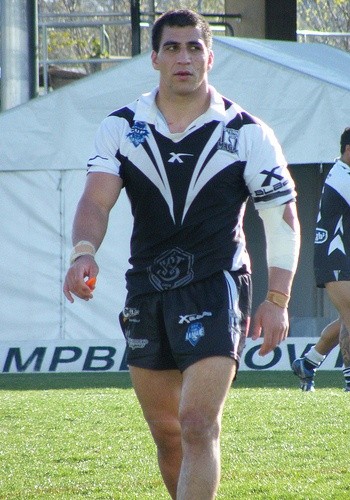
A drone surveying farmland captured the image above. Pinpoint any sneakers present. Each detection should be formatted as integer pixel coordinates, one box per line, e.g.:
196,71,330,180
291,356,318,392
344,376,350,390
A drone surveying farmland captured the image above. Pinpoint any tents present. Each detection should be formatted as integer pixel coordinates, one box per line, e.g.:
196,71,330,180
0,39,350,374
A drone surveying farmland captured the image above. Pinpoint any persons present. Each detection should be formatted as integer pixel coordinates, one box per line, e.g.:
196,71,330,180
291,129,350,392
63,9,301,499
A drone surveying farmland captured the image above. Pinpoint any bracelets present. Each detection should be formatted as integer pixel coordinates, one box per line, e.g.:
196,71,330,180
69,240,96,265
266,289,292,309
